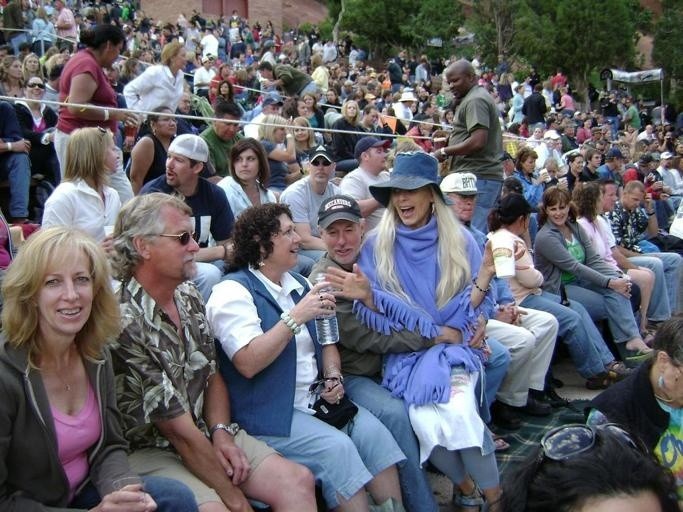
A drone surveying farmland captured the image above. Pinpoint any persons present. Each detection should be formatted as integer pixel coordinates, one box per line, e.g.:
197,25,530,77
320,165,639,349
0,0,683,512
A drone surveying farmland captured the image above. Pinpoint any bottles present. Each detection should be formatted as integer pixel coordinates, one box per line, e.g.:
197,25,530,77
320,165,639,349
314,272,339,346
124,122,137,152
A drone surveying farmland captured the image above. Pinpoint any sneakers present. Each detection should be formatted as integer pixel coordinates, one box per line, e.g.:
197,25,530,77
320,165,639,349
493,388,552,424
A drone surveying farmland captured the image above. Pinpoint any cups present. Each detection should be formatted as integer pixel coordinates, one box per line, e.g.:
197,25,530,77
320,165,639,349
490,235,516,279
113,477,146,505
104,225,113,237
539,168,552,183
558,177,566,181
433,138,446,151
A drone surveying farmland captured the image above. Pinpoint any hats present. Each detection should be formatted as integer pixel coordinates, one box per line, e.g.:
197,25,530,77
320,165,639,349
606,148,626,161
264,39,281,48
354,137,391,159
638,151,673,163
439,172,478,196
398,92,418,102
318,195,361,229
574,112,581,116
591,128,604,134
544,129,561,139
202,54,213,63
168,134,209,163
309,143,335,163
263,98,283,107
369,152,456,208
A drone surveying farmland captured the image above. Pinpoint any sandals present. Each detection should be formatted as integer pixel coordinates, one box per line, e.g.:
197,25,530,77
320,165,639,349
491,435,511,453
587,331,654,390
455,483,505,512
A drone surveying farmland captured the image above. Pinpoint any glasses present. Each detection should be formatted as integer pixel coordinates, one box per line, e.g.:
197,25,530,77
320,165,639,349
98,126,106,145
536,423,649,470
27,83,45,89
311,160,331,166
146,232,197,245
308,377,339,394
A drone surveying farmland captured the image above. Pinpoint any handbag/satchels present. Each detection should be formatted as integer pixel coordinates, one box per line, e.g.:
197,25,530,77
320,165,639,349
312,396,358,429
28,180,55,222
